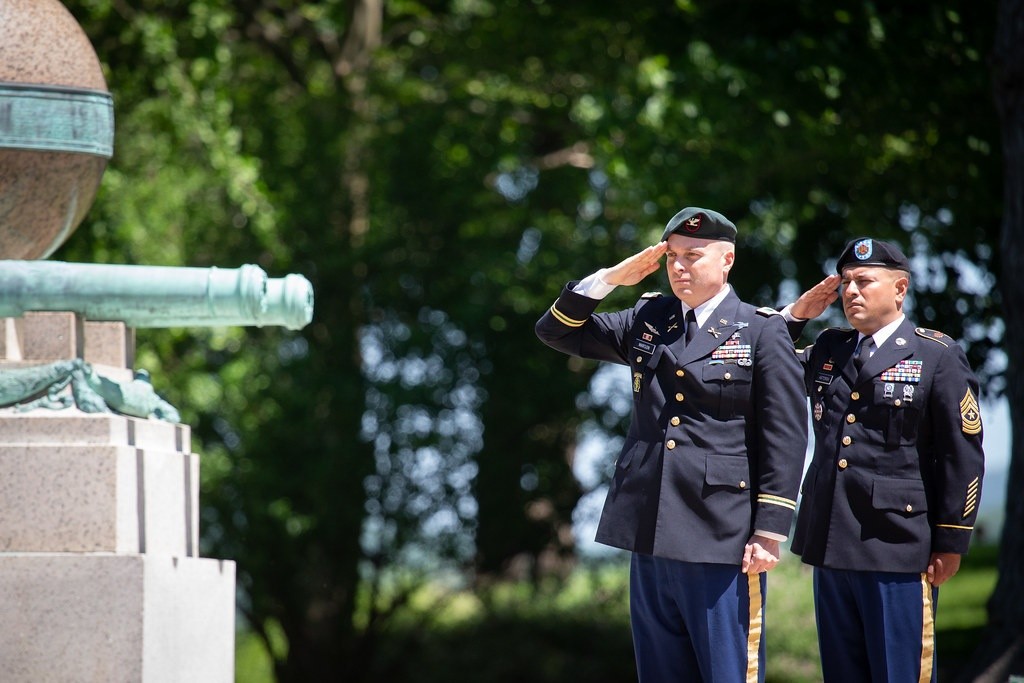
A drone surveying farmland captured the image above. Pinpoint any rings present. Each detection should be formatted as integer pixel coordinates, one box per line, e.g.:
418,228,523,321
764,568,768,571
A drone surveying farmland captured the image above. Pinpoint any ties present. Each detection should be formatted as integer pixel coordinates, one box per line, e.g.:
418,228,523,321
685,308,698,347
856,335,875,373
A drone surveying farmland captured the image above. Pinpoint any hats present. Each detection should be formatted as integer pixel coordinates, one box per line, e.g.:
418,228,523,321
836,237,912,277
660,206,738,242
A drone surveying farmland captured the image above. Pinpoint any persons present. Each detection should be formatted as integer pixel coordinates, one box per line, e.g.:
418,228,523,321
774,238,984,683
533,208,810,683
